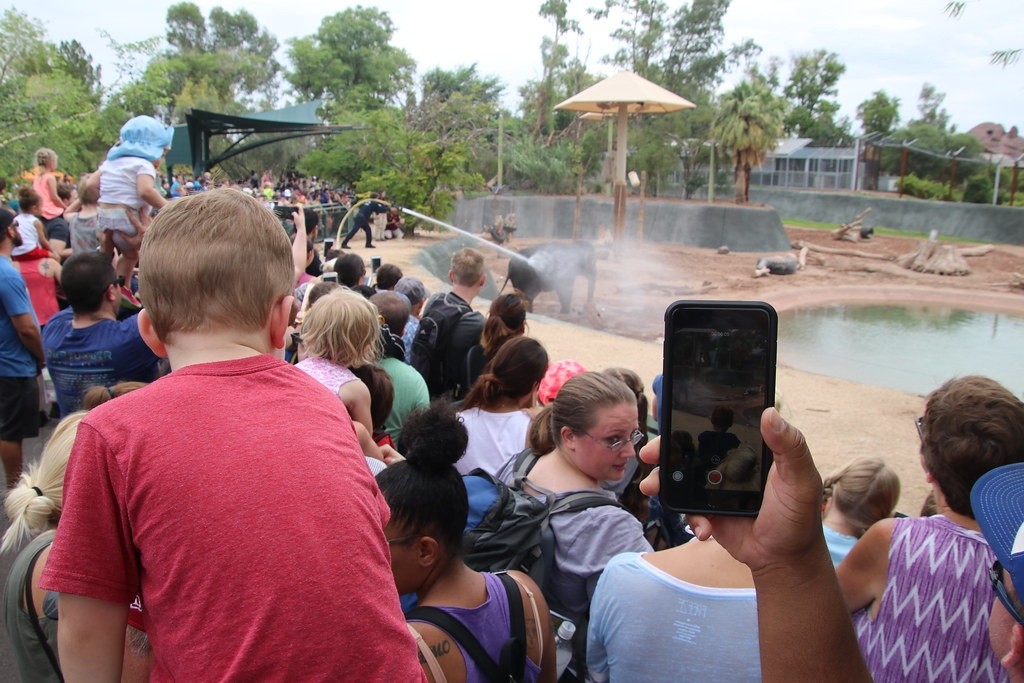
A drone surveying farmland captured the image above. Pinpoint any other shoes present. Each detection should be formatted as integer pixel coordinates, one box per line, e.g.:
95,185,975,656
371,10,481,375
39,409,48,428
341,243,351,249
49,401,60,419
365,243,375,248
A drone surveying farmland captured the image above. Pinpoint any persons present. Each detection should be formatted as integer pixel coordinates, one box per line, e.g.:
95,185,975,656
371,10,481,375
835,374,1024,683
586,534,762,683
775,394,783,412
643,495,696,549
0,116,556,683
653,374,662,417
921,489,938,516
970,462,1024,683
821,457,900,569
672,406,762,490
497,361,655,680
640,408,874,683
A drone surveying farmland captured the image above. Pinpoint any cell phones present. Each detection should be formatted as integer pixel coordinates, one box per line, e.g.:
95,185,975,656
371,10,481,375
372,256,381,276
323,271,338,284
324,239,334,257
657,299,779,517
274,206,299,220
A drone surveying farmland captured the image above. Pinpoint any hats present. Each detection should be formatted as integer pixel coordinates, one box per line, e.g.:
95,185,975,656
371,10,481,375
969,462,1024,602
394,276,431,304
0,208,16,232
106,114,174,160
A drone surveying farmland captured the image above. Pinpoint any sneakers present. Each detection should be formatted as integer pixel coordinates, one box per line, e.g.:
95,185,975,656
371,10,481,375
119,285,142,309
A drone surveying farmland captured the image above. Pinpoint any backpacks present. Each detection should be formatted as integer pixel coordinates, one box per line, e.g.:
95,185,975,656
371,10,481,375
409,293,470,389
459,446,629,573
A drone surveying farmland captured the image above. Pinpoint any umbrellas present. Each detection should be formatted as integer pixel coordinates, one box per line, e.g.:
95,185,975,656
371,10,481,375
554,71,697,247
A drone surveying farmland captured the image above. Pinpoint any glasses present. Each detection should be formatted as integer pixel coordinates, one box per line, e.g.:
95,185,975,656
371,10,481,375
576,427,644,452
915,416,926,446
9,221,19,228
989,559,1024,628
105,274,125,292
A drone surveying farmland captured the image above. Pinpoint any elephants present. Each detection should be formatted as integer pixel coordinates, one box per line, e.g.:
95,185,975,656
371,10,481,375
498,239,598,316
